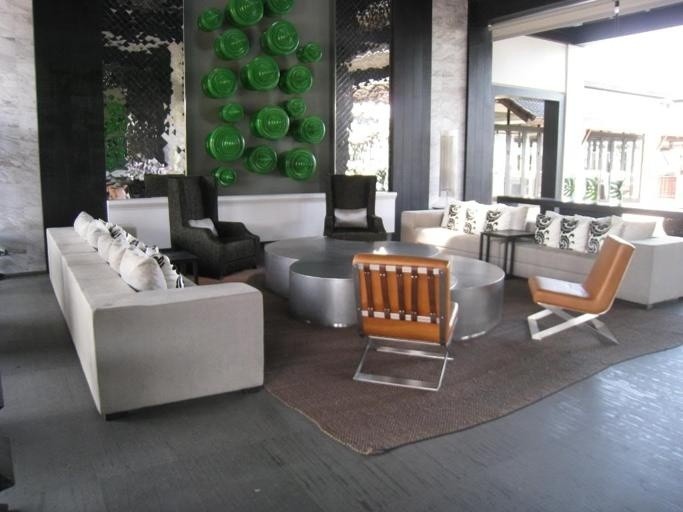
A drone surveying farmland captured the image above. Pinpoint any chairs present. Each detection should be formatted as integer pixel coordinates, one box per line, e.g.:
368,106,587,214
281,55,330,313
129,175,185,198
168,176,260,280
352,254,459,391
528,235,637,341
324,174,387,242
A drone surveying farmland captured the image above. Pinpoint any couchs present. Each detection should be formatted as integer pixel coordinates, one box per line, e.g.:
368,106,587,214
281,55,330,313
400,195,683,309
45,226,267,420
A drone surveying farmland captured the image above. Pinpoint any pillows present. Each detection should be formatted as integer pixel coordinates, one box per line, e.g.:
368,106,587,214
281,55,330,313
188,217,219,238
73,211,185,292
335,207,368,229
441,198,655,254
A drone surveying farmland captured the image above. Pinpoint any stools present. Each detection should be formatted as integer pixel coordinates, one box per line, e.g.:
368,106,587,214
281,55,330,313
165,251,202,284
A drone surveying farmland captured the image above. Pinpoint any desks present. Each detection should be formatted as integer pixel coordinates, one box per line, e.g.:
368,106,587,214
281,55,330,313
478,229,533,279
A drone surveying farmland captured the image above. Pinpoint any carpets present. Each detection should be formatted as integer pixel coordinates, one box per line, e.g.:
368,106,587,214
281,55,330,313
180,254,683,457
